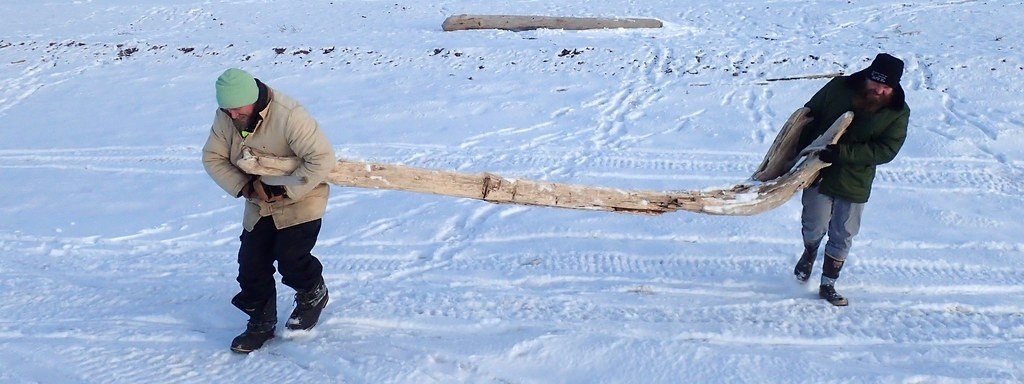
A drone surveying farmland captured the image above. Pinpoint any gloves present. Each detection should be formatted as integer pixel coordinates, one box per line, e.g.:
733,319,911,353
242,180,287,202
819,144,840,163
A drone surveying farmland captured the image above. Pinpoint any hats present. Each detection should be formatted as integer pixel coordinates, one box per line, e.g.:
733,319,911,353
866,53,904,90
215,68,259,108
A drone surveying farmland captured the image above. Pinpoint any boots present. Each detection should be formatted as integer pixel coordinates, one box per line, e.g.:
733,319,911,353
230,290,278,352
793,229,826,280
820,252,847,305
281,276,330,330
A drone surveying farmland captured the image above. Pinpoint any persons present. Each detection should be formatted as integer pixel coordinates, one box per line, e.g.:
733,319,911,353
793,53,910,306
202,68,335,354
